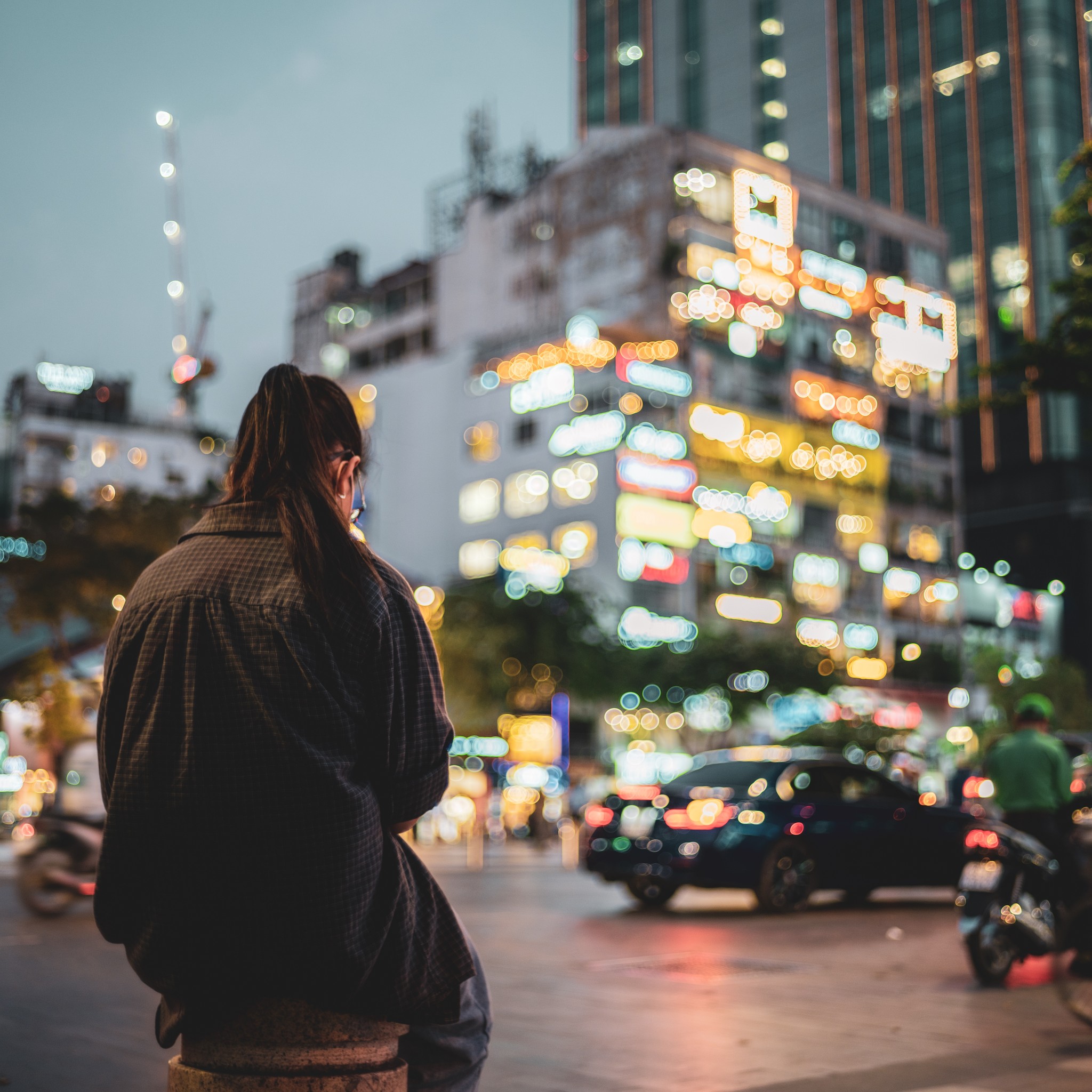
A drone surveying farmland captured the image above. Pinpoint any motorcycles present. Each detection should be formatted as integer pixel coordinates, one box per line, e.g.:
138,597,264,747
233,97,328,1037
952,814,1062,990
17,811,102,921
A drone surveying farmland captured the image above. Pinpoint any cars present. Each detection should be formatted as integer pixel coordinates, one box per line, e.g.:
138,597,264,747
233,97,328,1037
572,744,972,916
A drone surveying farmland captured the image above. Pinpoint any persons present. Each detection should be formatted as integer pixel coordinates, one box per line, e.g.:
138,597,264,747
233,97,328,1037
94,364,491,1092
989,693,1092,980
527,789,545,842
947,759,972,813
60,709,110,830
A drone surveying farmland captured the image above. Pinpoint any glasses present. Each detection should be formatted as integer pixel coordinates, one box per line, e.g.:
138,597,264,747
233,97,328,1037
330,449,366,524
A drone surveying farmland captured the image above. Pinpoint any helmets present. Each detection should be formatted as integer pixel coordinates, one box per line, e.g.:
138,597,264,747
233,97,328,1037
1015,694,1053,721
